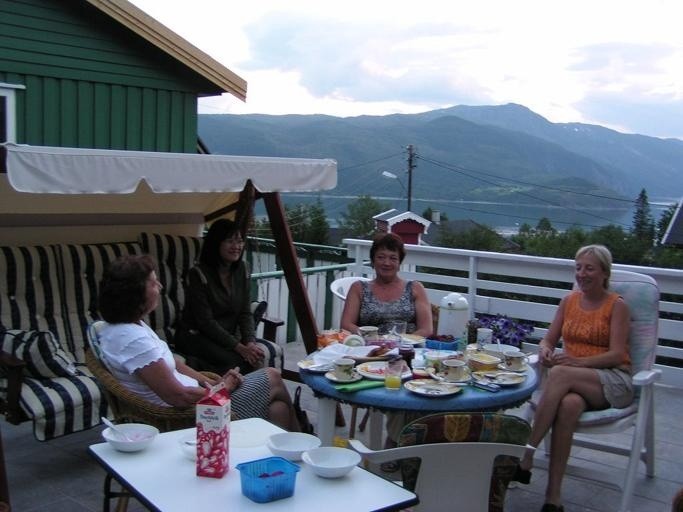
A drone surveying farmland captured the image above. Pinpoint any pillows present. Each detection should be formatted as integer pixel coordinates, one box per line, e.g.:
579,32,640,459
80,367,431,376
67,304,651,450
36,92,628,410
4,331,80,379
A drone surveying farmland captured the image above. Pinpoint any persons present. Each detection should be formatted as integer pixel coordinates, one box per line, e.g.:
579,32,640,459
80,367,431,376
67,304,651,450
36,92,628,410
174,218,285,375
511,244,634,512
98,256,300,434
340,234,433,473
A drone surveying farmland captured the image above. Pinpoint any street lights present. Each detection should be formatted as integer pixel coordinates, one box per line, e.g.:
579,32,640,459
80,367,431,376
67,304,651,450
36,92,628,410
382,170,412,211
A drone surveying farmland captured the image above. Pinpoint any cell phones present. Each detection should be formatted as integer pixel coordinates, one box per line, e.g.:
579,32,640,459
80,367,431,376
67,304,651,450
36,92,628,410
473,380,502,393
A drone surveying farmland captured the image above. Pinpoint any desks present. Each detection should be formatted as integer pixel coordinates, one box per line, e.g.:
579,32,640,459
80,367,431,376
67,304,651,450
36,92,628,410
87,417,418,512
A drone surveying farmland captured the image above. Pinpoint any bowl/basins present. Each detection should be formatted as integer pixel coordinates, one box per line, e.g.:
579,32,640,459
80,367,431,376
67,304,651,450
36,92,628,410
180,433,197,461
424,352,447,372
468,353,502,372
267,432,322,461
101,423,159,452
301,447,362,479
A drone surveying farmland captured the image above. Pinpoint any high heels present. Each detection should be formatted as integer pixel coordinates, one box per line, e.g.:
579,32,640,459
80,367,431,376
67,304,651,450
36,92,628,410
539,503,564,512
512,462,532,485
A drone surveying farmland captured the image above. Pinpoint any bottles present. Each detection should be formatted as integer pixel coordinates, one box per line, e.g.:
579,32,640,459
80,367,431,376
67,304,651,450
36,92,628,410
399,340,415,370
196,381,232,479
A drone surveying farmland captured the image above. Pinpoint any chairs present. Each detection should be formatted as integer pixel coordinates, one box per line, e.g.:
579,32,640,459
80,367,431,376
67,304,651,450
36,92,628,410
327,275,418,336
527,269,663,509
346,440,533,512
84,344,234,512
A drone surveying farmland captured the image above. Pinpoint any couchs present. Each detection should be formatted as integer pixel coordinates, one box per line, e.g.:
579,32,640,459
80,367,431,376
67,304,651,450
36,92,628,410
0,233,281,442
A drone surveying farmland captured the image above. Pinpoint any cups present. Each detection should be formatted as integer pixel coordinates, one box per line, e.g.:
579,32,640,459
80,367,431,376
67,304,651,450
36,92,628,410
384,364,403,391
476,328,493,351
503,350,529,371
393,322,406,344
358,326,378,339
443,360,469,381
328,359,356,380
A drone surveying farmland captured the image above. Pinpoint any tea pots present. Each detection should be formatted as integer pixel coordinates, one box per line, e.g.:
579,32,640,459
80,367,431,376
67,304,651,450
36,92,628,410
438,293,469,337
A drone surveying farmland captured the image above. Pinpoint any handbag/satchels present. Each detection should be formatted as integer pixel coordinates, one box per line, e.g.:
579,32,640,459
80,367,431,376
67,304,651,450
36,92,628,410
292,386,315,435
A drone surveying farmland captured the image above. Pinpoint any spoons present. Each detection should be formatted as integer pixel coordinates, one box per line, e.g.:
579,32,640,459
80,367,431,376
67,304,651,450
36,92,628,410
483,373,527,379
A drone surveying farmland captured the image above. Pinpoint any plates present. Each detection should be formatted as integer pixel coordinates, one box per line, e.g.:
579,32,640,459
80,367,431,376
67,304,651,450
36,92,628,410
472,370,526,386
482,344,520,352
297,359,330,372
325,372,363,383
357,362,411,379
404,379,462,396
